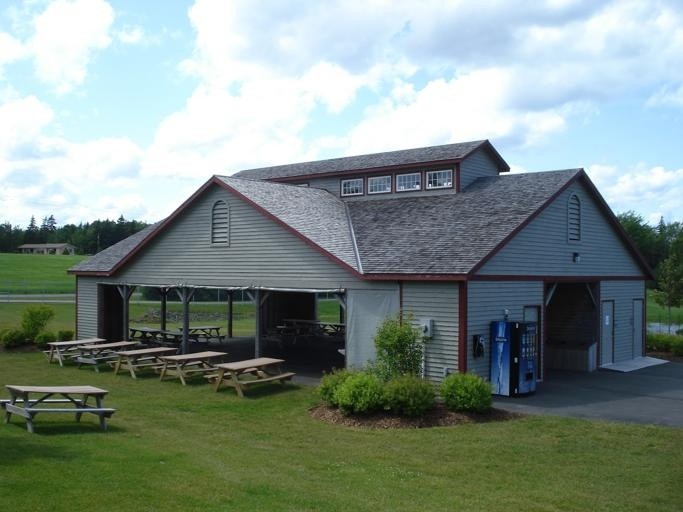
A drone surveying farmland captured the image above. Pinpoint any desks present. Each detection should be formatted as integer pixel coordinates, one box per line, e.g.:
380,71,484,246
4,385,109,433
77,341,139,373
212,357,285,397
159,350,228,384
114,347,179,378
47,337,106,367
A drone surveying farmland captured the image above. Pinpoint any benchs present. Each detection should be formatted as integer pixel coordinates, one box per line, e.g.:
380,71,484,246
129,328,226,349
183,368,220,372
152,361,203,369
1,398,84,421
239,372,297,386
203,368,258,379
26,407,119,433
106,356,155,364
131,362,173,366
92,355,120,360
42,346,117,357
